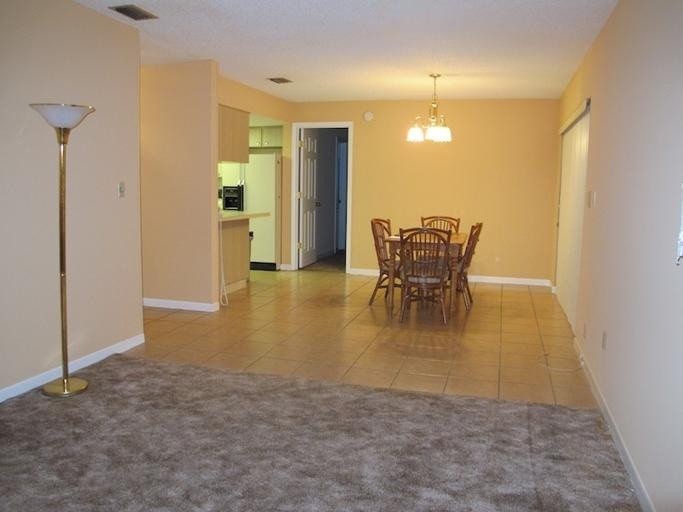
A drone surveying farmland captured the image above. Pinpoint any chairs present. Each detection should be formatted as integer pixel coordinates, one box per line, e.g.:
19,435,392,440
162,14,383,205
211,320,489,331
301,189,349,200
368,216,483,325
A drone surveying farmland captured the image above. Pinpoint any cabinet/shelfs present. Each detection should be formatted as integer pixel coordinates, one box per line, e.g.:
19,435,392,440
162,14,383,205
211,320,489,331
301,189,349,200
249,126,282,149
219,219,249,296
218,104,249,164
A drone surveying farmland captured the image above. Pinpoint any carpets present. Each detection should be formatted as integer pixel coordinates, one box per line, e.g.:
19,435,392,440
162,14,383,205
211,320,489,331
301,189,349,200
0,352,644,510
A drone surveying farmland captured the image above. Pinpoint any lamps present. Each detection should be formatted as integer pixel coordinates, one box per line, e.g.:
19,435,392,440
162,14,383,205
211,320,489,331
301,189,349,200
25,100,96,399
406,73,451,143
